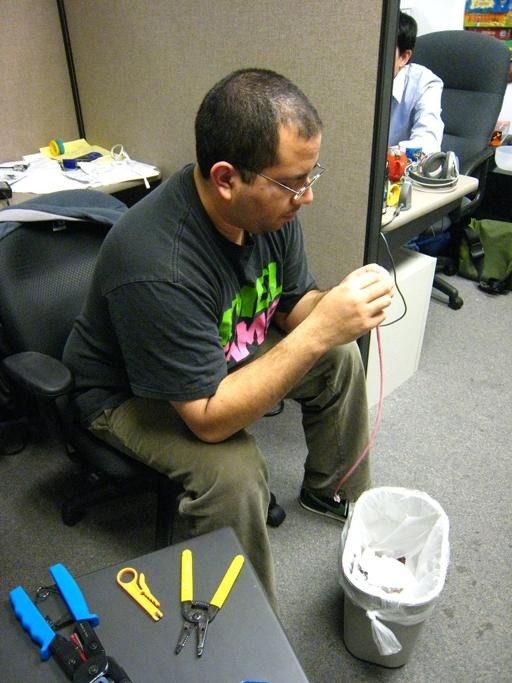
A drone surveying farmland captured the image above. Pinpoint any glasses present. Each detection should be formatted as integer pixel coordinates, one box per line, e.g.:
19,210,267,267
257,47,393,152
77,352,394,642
244,163,326,201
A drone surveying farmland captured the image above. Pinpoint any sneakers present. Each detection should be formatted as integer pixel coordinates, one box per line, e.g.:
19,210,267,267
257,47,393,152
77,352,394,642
299,488,349,522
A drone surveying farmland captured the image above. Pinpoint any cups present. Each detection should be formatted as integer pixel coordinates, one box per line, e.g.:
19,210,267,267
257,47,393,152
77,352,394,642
405,145,423,163
387,148,407,181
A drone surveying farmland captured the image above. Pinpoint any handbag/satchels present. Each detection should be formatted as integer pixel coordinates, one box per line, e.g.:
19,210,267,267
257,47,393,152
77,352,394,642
458,218,511,296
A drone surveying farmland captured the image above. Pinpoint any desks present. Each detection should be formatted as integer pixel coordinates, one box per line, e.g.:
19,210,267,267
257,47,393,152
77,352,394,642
0,142,161,192
379,173,479,260
1,522,314,681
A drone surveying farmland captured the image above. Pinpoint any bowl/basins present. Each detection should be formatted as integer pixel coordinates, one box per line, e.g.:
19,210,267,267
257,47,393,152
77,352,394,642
495,145,512,170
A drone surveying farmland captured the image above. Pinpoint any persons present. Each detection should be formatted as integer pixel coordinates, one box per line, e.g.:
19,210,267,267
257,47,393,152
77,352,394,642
63,68,394,619
387,11,445,251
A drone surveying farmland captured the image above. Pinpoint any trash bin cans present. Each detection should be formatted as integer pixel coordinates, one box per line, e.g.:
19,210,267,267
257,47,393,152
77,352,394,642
341,486,450,667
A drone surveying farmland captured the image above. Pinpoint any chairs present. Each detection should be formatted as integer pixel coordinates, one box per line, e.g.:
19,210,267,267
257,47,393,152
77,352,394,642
0,187,287,547
404,28,512,310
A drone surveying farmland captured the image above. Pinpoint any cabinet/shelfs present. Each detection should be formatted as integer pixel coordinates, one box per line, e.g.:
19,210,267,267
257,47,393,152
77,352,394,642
367,246,438,410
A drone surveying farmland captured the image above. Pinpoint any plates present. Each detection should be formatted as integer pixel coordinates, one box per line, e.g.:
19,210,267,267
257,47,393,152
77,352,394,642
405,164,457,194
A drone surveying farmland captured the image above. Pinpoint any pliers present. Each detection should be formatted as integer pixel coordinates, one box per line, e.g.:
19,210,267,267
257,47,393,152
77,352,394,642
175,549,245,657
8,562,132,683
